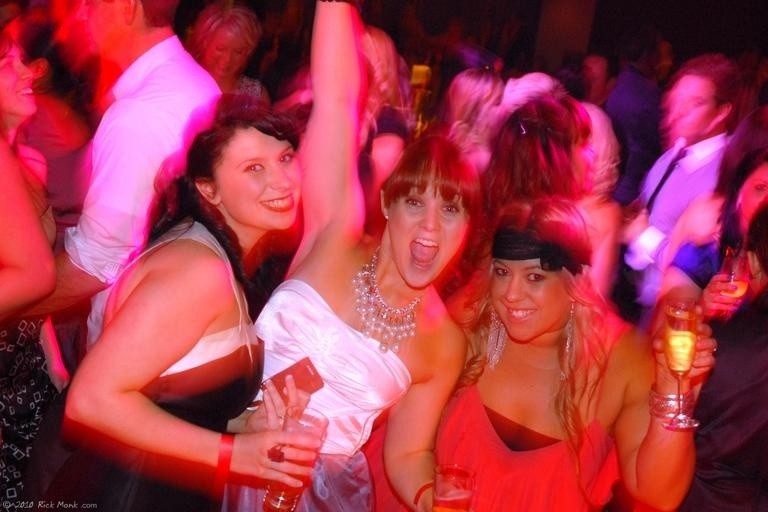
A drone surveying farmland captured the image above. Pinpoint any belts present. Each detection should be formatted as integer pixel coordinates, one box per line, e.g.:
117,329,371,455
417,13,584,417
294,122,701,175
661,305,699,432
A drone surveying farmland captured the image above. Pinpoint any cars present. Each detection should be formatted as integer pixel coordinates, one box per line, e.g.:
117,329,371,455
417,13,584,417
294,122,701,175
719,255,749,298
431,464,477,512
261,406,330,512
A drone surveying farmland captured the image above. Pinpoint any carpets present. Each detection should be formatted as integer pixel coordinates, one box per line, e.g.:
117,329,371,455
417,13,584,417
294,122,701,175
645,148,686,213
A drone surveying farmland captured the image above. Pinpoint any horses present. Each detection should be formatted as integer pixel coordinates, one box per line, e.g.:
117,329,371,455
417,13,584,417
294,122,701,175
260,357,324,409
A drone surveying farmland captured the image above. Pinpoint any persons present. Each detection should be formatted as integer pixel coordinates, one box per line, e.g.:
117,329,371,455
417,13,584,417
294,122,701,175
1,1,767,510
